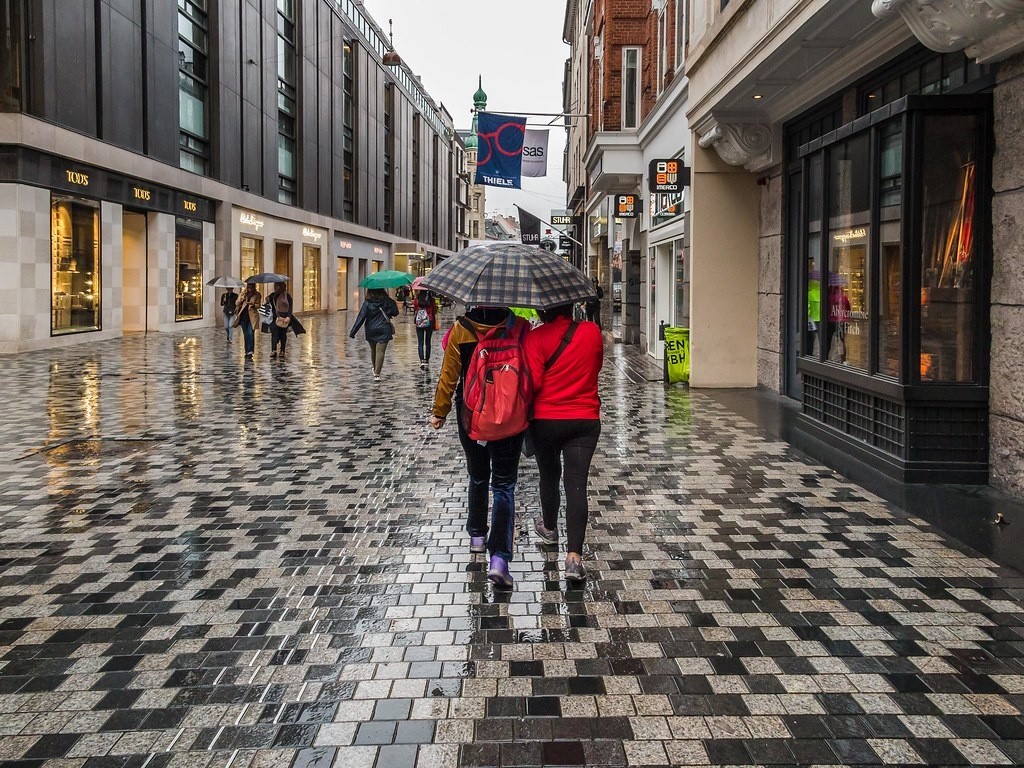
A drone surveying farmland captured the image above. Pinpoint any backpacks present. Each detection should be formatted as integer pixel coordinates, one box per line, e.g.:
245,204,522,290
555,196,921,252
456,314,532,442
416,307,430,328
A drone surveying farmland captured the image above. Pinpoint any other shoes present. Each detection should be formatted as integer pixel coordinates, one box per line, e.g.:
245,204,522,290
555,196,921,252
371,366,381,381
419,359,430,367
270,350,277,357
226,338,233,344
279,351,285,357
245,352,254,359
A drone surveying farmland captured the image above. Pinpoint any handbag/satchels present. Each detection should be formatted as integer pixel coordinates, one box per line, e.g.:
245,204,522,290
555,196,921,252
275,318,289,329
258,300,274,324
520,426,537,457
388,322,396,335
230,313,239,328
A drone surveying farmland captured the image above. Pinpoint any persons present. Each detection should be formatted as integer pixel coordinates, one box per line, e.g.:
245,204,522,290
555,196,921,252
525,304,608,582
807,281,821,357
431,303,533,586
265,281,294,357
349,288,399,381
219,287,238,344
579,275,604,332
825,286,851,366
413,290,437,368
235,283,262,358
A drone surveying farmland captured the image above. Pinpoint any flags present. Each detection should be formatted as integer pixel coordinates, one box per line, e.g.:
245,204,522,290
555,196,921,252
520,127,550,178
473,111,528,189
516,206,541,246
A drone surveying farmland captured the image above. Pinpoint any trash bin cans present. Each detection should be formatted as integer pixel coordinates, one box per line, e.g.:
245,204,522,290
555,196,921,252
663,327,690,384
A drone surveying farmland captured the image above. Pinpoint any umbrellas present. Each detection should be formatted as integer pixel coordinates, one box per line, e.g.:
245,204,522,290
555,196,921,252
245,273,291,305
807,270,849,287
204,275,249,302
411,276,429,291
418,243,599,318
358,269,414,297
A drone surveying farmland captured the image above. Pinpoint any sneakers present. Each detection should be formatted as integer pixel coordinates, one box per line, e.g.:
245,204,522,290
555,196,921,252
469,534,488,552
487,557,515,588
564,556,587,580
533,517,559,544
